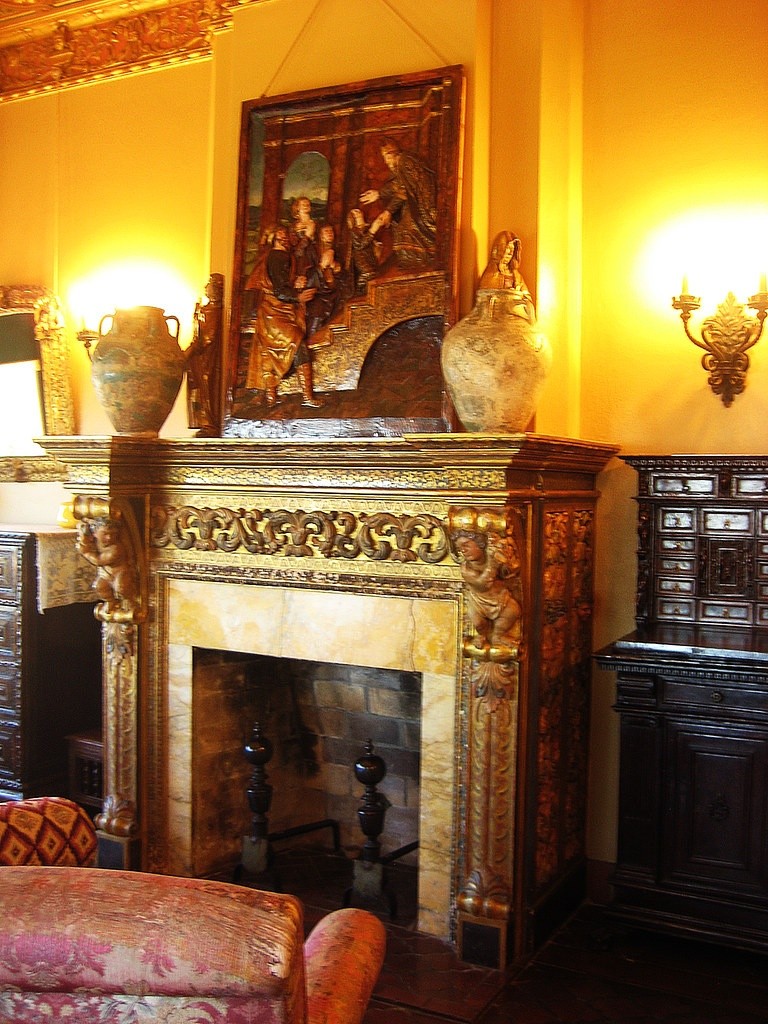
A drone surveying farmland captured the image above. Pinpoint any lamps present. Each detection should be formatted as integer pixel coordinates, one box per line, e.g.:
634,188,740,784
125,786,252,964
670,272,767,411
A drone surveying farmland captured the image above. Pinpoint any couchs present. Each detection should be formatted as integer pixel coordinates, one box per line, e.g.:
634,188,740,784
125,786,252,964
0,796,385,1024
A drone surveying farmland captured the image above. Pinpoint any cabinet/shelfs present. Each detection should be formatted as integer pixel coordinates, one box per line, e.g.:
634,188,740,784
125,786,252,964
591,456,768,950
0,531,102,799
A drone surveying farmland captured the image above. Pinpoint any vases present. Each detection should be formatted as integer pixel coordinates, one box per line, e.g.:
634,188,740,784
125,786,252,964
90,305,185,437
437,288,555,434
